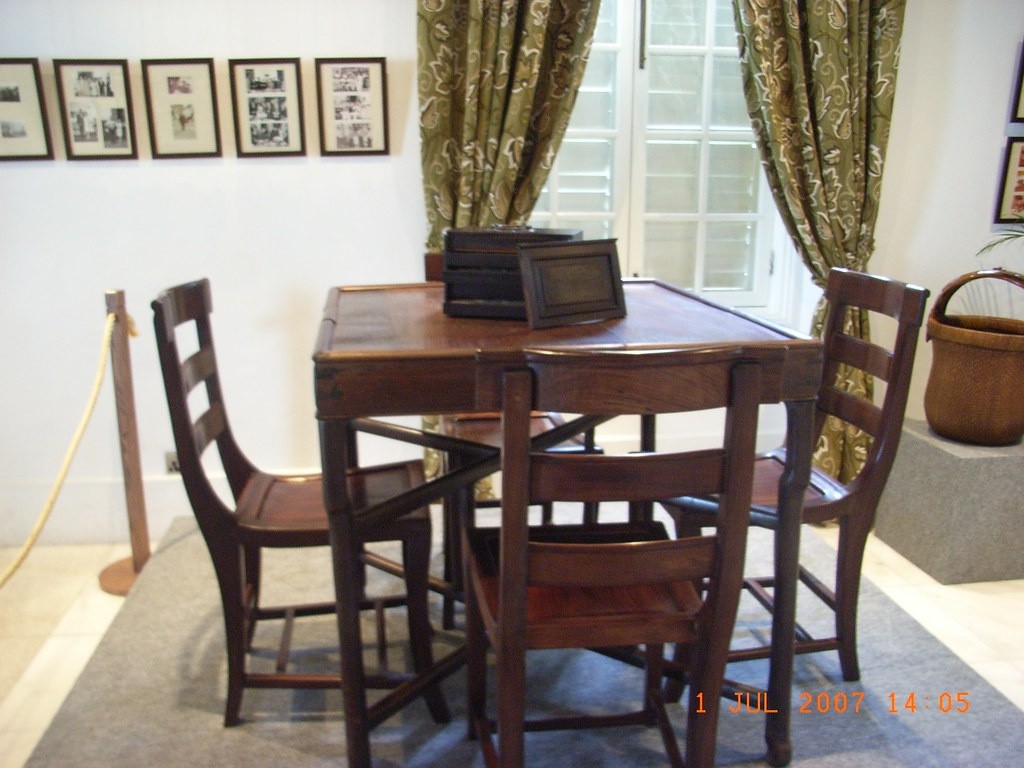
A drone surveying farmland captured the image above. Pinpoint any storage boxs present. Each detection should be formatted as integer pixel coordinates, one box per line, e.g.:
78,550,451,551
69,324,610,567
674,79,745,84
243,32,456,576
440,225,583,320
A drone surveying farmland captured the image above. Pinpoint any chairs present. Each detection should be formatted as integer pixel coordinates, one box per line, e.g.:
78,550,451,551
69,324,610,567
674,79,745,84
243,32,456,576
150,278,453,726
459,342,788,768
442,411,604,630
661,267,930,704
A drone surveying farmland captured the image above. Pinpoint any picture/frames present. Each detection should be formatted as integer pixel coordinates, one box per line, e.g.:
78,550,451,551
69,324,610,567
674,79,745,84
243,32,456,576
228,57,307,158
521,237,628,330
141,58,223,158
993,136,1024,224
53,59,139,161
315,57,390,157
1010,43,1024,123
0,57,54,161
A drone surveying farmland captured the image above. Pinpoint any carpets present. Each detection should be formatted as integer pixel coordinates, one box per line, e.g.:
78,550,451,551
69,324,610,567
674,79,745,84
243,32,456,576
23,515,1024,768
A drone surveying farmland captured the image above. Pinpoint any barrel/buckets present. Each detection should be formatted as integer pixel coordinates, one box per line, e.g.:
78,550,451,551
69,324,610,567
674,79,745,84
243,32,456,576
926,268,1024,444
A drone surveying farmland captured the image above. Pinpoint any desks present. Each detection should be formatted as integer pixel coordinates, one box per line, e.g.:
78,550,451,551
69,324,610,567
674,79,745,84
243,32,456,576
312,277,826,768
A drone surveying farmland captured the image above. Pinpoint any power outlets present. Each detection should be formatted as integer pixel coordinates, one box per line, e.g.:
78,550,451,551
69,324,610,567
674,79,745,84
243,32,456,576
165,451,181,474
